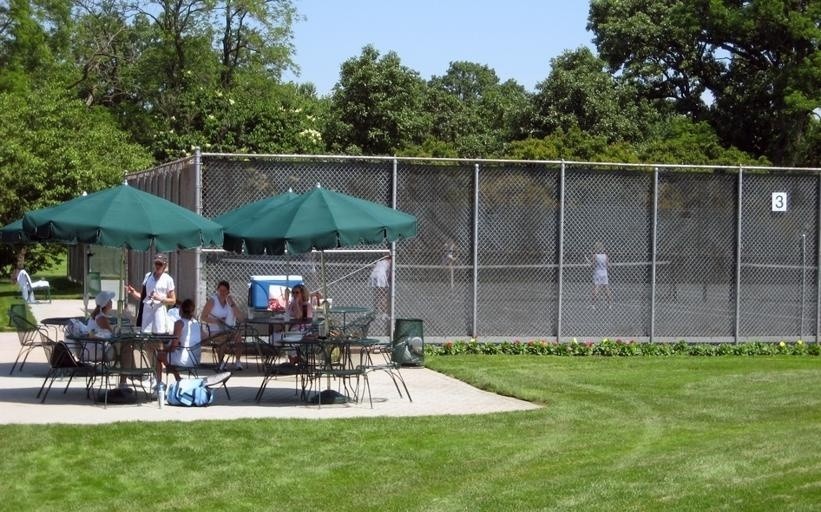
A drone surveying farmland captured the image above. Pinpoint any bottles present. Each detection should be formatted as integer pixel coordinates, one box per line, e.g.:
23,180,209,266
310,289,330,320
301,305,307,320
299,322,352,340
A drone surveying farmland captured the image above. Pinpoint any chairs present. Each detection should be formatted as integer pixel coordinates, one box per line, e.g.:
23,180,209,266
17,269,51,303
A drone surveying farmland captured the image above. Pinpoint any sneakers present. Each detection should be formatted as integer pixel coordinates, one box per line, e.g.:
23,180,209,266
234,362,244,371
119,374,166,399
219,360,225,370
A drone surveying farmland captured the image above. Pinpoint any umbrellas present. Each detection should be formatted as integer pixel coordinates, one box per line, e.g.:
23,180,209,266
22,169,223,330
223,181,417,391
0,190,90,318
213,187,300,363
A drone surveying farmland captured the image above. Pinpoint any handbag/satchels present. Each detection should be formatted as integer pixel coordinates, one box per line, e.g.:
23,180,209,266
136,271,152,328
164,376,214,406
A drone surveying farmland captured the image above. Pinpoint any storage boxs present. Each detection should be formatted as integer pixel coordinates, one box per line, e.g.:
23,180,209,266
247,274,303,309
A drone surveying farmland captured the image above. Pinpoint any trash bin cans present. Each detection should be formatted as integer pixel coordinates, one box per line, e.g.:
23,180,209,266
87,272,101,299
391,318,424,366
9,304,26,327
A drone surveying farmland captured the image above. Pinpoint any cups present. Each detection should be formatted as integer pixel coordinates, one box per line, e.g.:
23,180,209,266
247,307,253,320
87,329,94,336
110,325,140,338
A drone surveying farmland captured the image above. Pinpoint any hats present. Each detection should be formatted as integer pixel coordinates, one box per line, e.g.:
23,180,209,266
95,290,116,309
153,253,168,265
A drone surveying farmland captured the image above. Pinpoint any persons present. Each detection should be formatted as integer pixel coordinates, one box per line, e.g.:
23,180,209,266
122,253,177,388
439,237,458,299
76,292,148,393
588,241,611,308
150,298,201,391
283,284,312,369
366,258,390,320
198,280,244,372
